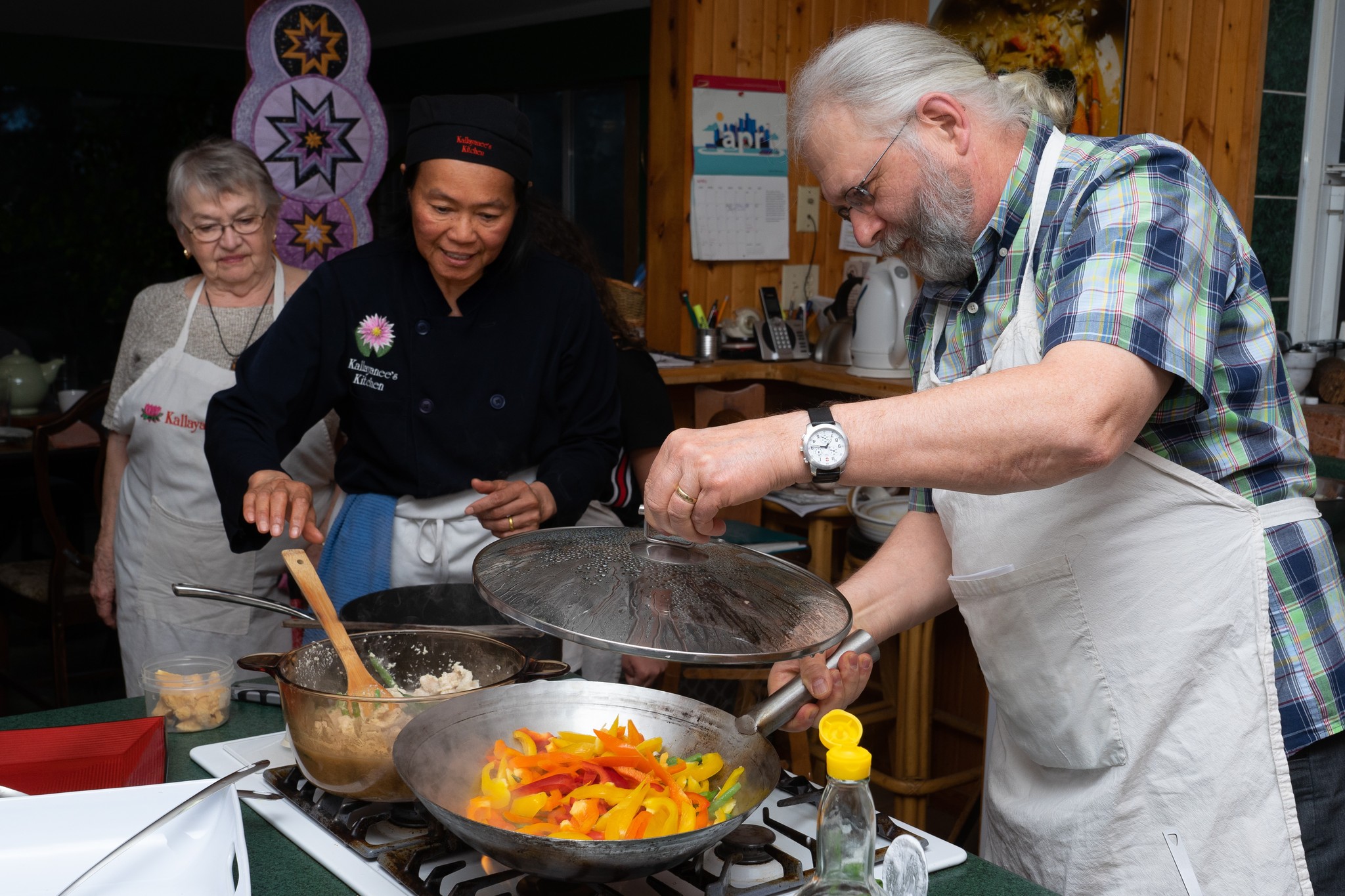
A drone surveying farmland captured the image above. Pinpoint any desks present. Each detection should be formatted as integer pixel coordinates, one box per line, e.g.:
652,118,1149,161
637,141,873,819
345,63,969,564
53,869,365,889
651,346,917,398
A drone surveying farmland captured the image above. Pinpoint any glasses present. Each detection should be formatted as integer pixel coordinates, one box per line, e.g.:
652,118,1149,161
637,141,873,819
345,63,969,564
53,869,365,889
835,112,914,225
175,203,270,243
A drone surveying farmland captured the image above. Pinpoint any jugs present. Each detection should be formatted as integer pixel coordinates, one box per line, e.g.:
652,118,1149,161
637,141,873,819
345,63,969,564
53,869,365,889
0,349,64,415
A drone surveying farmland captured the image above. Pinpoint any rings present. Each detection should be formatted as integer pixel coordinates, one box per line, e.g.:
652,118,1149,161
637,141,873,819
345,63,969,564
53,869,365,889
508,516,514,531
676,484,697,504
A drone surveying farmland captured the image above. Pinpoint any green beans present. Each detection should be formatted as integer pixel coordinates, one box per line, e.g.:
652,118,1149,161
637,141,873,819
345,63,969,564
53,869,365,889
338,655,444,719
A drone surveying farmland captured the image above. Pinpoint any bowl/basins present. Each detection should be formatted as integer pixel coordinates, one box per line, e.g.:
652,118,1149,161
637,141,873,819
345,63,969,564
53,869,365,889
851,485,910,543
1283,352,1317,396
138,650,235,732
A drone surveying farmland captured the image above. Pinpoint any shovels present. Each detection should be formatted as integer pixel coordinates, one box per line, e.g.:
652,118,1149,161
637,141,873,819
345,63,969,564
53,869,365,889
281,545,399,714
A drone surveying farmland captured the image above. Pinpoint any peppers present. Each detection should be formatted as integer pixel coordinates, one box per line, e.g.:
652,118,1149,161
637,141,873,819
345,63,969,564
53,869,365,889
466,717,743,842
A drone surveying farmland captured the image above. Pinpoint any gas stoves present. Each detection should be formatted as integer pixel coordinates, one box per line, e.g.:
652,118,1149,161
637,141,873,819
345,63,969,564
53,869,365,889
187,726,970,896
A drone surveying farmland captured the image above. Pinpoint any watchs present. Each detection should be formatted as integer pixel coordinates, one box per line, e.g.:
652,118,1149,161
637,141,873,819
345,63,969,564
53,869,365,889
800,406,849,484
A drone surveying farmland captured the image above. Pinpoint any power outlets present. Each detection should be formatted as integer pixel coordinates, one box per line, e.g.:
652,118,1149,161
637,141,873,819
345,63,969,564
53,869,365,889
797,184,821,233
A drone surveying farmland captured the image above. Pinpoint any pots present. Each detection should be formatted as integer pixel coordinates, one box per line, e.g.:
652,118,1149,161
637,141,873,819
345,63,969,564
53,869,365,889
170,582,565,668
239,628,572,805
391,628,879,875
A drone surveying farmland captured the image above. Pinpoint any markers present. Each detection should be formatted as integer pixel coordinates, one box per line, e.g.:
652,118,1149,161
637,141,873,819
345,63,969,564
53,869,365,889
693,304,709,331
710,312,718,330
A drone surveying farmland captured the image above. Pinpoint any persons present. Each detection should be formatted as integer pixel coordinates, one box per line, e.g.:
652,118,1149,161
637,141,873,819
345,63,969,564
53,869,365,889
91,141,342,698
650,22,1345,896
204,94,677,688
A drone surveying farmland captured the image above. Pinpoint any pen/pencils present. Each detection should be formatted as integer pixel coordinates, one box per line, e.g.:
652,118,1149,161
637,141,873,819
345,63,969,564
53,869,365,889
803,295,812,321
684,293,698,328
706,298,718,323
716,294,729,328
788,296,795,320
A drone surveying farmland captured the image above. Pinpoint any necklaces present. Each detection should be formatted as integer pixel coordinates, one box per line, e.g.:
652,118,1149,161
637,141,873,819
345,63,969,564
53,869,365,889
205,283,275,371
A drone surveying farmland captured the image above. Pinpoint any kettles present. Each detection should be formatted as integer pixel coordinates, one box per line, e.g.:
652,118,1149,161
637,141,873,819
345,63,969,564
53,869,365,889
846,254,920,379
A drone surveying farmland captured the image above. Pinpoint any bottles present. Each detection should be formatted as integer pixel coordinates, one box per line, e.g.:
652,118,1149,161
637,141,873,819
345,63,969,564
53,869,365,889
788,709,890,896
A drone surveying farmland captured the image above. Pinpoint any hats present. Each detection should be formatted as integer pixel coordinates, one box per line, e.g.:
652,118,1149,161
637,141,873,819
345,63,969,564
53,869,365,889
406,94,534,191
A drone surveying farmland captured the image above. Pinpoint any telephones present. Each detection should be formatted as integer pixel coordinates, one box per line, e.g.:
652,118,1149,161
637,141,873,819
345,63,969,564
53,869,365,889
754,283,813,361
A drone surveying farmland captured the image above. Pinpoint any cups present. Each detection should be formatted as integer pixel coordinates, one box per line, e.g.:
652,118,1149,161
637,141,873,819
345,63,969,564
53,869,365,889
58,390,87,412
693,326,723,363
